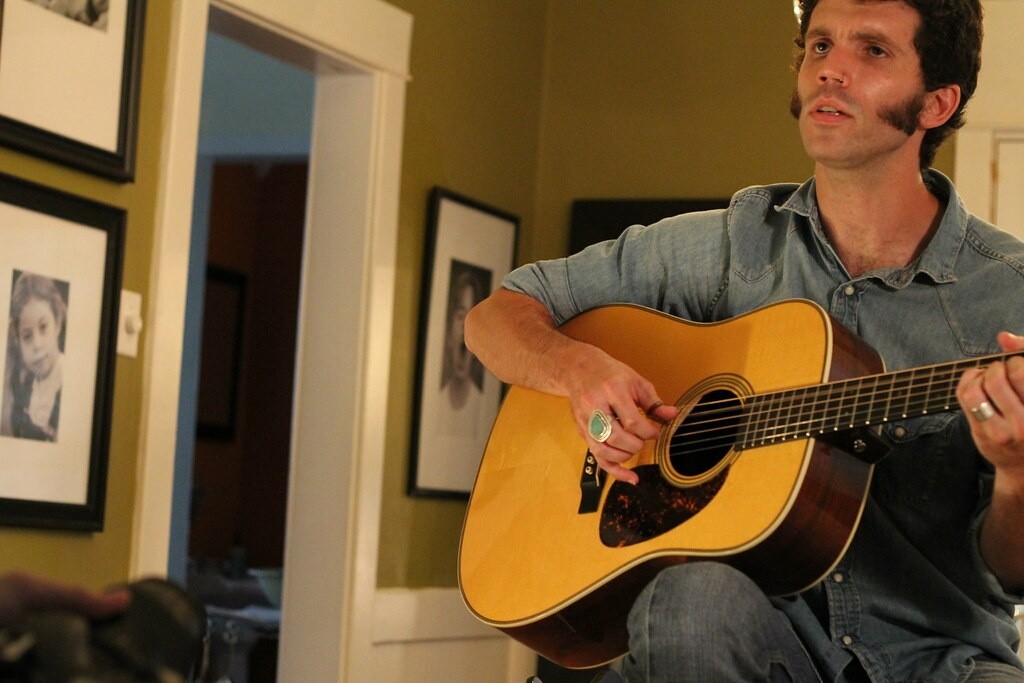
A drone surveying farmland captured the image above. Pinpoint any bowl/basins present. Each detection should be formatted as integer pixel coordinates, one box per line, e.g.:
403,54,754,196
248,569,281,608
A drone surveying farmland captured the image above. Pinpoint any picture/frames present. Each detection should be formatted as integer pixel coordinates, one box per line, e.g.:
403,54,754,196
405,186,522,502
196,264,249,443
0,0,148,185
0,171,128,534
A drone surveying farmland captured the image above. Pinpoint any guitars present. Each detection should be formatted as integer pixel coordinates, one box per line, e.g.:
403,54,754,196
455,297,1023,670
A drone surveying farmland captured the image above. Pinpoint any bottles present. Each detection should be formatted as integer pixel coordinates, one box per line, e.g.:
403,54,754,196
227,532,248,595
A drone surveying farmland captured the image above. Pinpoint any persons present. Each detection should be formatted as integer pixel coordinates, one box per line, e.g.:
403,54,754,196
464,0,1024,683
436,276,490,437
5,271,71,441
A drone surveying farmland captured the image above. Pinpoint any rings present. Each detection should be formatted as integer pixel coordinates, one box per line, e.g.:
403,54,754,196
587,409,618,442
646,400,666,415
970,401,995,422
